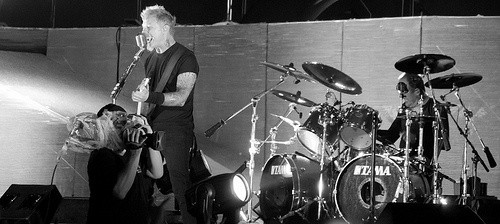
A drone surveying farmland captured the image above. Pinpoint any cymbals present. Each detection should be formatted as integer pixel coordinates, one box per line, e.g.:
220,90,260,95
395,114,446,120
301,62,362,95
394,54,456,75
269,89,317,108
425,73,483,90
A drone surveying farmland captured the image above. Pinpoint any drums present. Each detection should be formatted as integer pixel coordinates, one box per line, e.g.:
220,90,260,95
258,152,338,224
296,104,343,158
337,103,381,151
332,153,430,224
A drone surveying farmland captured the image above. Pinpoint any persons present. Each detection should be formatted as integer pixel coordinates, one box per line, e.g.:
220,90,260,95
376,73,451,202
129,5,203,224
84,104,164,224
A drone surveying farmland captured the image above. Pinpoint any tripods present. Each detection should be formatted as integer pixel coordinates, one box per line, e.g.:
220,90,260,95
274,100,337,224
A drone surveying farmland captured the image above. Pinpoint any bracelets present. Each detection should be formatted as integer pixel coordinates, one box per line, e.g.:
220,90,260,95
144,91,165,105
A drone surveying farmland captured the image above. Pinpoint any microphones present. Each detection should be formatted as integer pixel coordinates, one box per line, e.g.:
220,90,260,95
134,34,146,60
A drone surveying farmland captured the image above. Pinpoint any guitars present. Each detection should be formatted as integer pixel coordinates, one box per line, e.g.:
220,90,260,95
128,77,151,125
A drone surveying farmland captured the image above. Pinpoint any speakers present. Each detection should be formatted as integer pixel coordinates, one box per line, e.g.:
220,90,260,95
0,183,90,224
373,202,486,224
